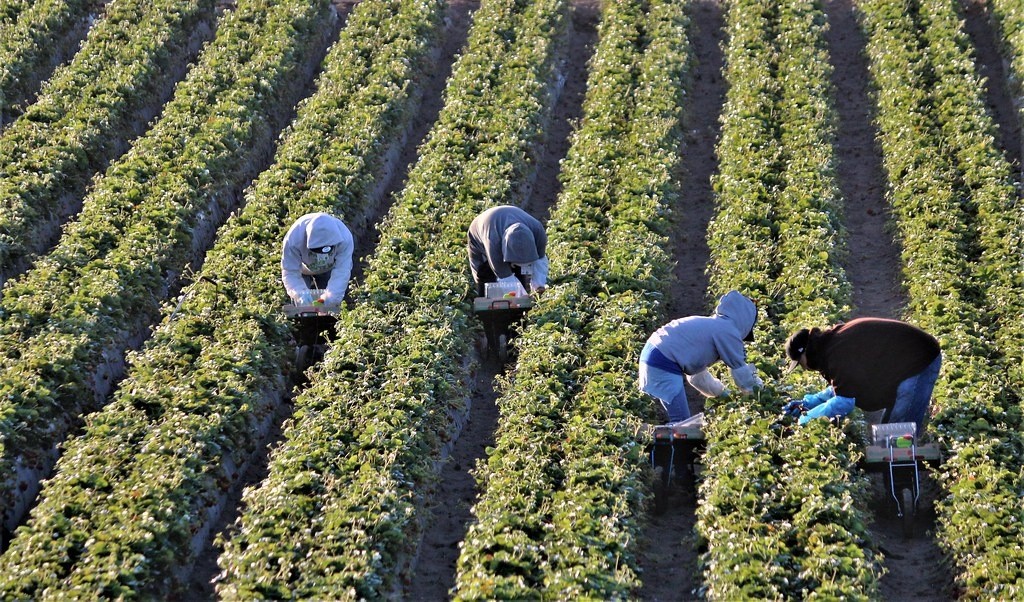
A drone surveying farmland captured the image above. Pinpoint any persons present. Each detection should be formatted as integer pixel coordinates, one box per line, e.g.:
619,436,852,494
467,205,548,358
281,212,355,306
780,317,942,500
638,291,764,423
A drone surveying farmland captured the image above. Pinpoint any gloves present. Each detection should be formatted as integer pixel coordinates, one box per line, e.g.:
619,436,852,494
782,401,808,416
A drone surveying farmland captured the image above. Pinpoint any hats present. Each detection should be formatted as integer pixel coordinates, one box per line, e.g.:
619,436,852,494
786,329,810,374
